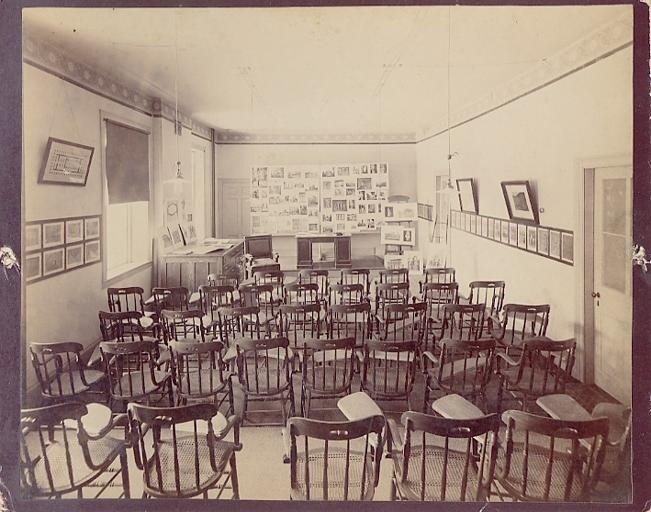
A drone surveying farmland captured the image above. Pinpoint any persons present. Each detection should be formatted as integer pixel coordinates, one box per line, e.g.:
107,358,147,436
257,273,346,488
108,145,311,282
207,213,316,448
336,181,347,197
404,232,412,241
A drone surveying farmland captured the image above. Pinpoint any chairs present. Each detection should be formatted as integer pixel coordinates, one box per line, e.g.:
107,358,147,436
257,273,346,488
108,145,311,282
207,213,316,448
498,336,578,414
98,333,174,426
27,341,109,442
424,335,496,419
358,337,422,426
301,337,356,419
245,233,282,281
167,341,236,422
387,405,502,503
128,400,245,502
485,408,613,502
90,271,572,376
21,400,131,503
277,413,387,503
234,337,296,429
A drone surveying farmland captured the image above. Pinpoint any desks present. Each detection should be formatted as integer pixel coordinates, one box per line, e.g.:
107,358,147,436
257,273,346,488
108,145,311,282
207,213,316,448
296,232,353,269
159,238,244,290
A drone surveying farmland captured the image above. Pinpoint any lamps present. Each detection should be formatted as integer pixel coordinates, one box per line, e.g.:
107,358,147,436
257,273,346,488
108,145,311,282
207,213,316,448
161,79,194,193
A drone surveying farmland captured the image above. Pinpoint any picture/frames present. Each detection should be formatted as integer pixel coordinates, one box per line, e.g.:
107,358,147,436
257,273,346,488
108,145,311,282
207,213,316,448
416,177,576,265
24,214,104,286
39,137,96,187
